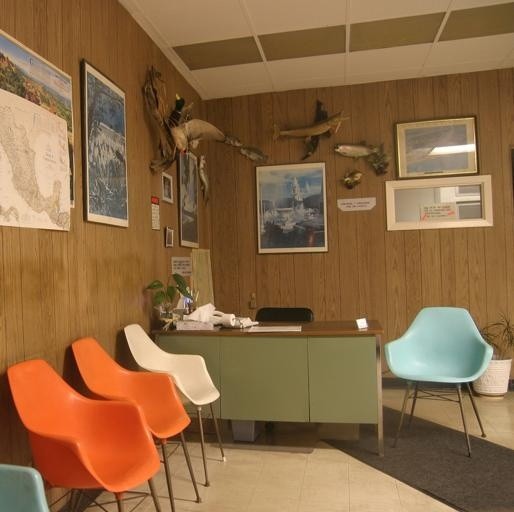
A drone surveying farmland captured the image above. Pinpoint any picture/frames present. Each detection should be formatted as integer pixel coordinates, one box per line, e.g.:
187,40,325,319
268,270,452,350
149,320,385,458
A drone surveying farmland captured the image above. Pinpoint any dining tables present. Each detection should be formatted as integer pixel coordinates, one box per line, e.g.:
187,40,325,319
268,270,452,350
144,272,194,330
471,310,514,397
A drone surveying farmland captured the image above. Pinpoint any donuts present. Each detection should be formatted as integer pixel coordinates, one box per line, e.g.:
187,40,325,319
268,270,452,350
164,93,226,156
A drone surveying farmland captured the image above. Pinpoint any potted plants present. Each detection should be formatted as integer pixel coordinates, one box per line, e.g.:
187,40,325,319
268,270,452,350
383,174,494,231
176,147,200,249
78,57,130,228
255,161,328,254
161,171,174,206
165,225,174,248
395,114,481,180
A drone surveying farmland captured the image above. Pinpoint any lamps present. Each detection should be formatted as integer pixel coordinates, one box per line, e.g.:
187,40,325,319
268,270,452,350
1,462,48,511
123,323,227,487
384,305,494,458
6,357,161,511
255,307,315,321
71,337,202,511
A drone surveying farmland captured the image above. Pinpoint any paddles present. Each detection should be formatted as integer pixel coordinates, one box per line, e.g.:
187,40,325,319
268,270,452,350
319,404,513,511
